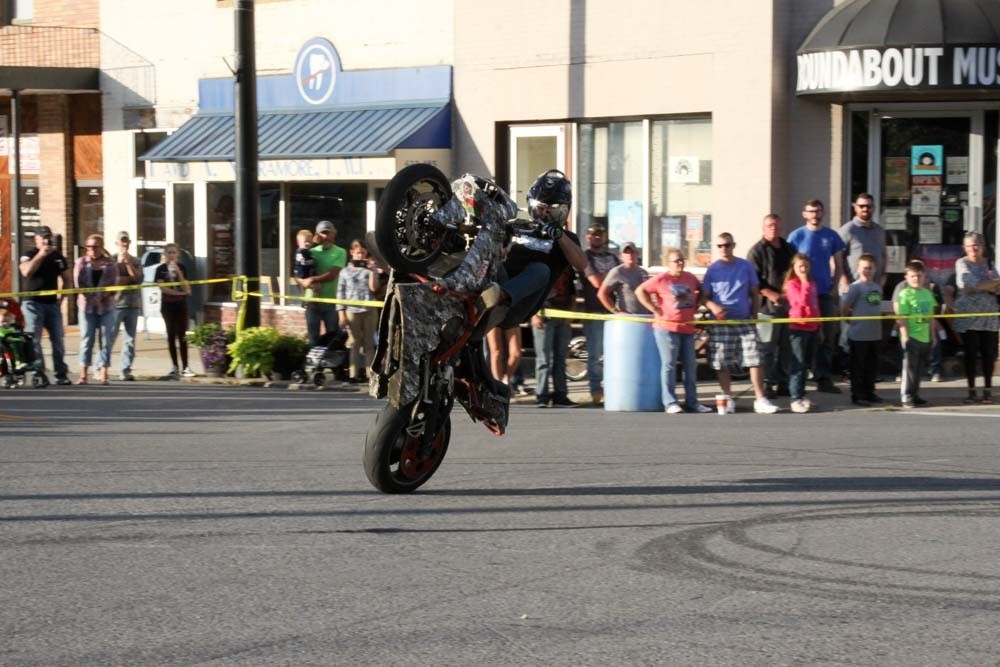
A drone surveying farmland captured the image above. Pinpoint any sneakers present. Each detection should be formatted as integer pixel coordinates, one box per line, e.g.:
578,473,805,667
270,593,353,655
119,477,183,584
180,367,196,377
169,366,178,376
553,397,580,407
538,401,549,407
500,378,534,397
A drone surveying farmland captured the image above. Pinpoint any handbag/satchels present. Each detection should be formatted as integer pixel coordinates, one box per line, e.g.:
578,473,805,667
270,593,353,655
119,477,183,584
755,310,782,344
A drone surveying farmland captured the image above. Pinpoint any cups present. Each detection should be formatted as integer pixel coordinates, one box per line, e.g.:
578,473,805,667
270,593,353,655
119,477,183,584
168,265,176,277
715,394,729,415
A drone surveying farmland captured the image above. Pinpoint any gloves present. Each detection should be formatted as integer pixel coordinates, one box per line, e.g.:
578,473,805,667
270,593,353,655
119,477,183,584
529,222,556,239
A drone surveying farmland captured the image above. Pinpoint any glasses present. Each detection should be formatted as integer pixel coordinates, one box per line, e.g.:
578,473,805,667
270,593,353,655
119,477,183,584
856,205,872,208
670,258,684,263
717,244,730,248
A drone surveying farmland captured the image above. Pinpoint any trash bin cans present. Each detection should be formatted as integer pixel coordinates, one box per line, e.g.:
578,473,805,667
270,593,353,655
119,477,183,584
603,314,664,412
139,248,194,317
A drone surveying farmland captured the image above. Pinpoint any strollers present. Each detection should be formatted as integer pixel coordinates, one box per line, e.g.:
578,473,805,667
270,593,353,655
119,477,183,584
0,296,50,390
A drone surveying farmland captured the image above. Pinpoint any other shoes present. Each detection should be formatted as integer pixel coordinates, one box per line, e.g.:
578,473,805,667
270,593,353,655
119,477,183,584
665,373,993,413
337,375,370,386
14,363,26,370
31,372,135,387
471,282,500,328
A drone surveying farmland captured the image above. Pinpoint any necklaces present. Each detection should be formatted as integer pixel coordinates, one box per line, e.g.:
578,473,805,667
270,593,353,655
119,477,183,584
92,255,103,260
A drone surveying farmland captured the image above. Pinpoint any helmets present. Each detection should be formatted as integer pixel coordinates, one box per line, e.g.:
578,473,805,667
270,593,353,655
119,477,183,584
526,168,572,226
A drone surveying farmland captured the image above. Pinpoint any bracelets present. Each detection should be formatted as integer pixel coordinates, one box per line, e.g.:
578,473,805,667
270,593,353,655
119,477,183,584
611,308,617,312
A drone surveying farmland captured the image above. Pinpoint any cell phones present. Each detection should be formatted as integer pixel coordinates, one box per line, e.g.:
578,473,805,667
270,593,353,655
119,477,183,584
353,260,367,268
47,239,53,246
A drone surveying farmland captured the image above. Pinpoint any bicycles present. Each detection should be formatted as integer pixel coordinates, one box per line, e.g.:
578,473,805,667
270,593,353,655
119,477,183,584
677,306,753,382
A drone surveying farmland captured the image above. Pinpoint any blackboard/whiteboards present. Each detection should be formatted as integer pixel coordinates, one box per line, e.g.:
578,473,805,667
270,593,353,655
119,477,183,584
259,276,274,305
277,276,303,306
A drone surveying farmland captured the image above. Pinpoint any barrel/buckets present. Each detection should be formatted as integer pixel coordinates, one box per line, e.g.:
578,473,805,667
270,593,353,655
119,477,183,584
602,314,661,412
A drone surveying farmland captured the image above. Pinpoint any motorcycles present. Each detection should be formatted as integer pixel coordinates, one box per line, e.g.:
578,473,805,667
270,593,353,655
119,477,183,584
362,163,563,494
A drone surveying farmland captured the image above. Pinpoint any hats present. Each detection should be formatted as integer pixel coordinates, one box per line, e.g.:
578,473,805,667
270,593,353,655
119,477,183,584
586,223,605,235
117,231,130,241
619,242,636,252
316,221,335,233
34,226,52,237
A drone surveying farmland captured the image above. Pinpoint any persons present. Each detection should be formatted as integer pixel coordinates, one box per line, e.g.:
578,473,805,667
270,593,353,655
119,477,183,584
472,169,589,328
295,220,389,387
0,225,197,388
486,192,1000,414
